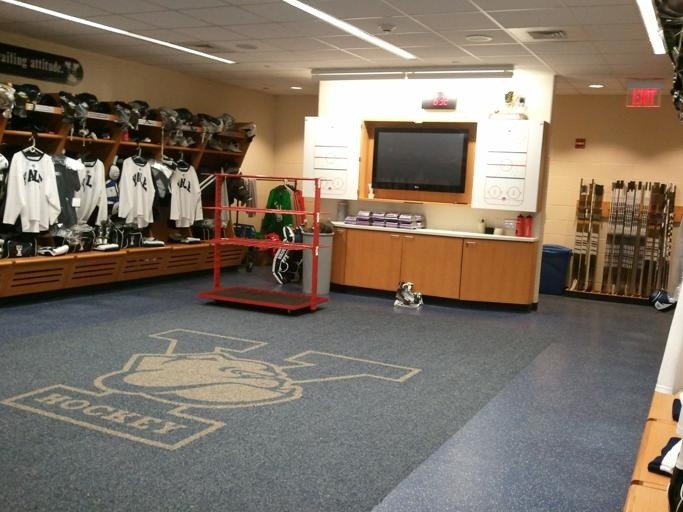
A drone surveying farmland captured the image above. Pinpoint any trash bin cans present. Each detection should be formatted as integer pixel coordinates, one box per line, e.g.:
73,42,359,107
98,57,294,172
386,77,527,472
302,232,335,295
540,244,573,295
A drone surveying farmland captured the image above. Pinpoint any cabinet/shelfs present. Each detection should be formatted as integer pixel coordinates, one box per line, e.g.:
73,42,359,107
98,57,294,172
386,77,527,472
344,228,463,301
0,103,252,298
193,172,332,313
330,227,346,286
460,238,538,305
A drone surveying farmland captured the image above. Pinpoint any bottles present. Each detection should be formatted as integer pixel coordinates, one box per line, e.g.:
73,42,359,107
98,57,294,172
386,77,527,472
517,214,531,237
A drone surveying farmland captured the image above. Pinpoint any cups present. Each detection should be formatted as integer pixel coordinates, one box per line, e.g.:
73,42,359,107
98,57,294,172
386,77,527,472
503,219,516,237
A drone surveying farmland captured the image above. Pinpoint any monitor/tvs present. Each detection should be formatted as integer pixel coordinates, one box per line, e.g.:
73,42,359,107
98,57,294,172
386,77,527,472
371,128,469,194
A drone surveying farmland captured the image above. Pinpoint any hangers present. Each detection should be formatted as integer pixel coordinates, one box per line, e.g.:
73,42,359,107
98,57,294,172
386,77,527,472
133,147,147,164
278,179,292,191
23,135,44,155
176,151,189,167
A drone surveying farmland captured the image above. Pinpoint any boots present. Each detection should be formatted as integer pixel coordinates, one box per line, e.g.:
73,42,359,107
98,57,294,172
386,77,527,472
394,281,423,304
